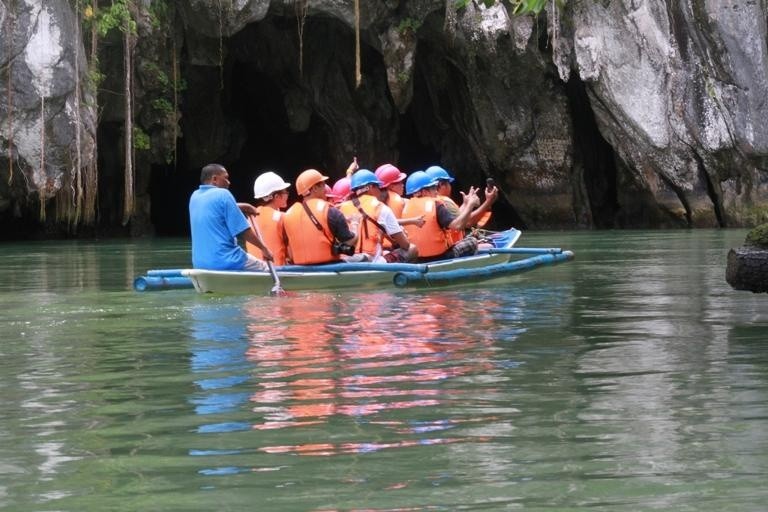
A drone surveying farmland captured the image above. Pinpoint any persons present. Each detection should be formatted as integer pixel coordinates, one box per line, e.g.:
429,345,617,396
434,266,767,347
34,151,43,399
189,163,274,272
325,161,500,263
281,168,372,266
245,171,292,266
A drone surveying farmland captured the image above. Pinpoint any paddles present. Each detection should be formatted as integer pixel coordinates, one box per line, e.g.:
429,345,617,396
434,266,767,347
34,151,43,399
244,208,287,296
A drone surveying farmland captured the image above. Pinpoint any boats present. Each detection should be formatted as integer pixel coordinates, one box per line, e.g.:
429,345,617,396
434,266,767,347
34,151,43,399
181,228,522,294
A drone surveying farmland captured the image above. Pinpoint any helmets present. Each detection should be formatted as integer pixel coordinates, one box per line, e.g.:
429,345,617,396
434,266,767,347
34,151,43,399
296,169,330,195
405,171,439,196
375,162,407,188
425,166,455,182
324,177,354,203
350,169,383,190
254,172,292,199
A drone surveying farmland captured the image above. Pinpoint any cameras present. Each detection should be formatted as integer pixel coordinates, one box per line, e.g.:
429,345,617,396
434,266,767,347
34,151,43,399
486,179,493,191
332,243,354,257
